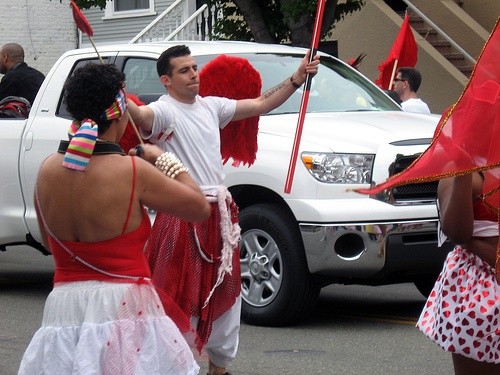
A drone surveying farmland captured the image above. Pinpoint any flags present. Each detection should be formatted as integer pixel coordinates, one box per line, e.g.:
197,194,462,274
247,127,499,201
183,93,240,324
375,15,418,91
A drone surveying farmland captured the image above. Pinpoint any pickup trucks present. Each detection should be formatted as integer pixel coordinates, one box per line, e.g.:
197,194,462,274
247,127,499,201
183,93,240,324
0,40,453,326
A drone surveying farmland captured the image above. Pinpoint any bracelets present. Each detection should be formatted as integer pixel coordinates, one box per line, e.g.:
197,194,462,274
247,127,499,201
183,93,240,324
290,75,302,88
154,151,190,179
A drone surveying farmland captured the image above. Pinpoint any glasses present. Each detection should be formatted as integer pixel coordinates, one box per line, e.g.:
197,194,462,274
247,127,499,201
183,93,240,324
395,78,411,84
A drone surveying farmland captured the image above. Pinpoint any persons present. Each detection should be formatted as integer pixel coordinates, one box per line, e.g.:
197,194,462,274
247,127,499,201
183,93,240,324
127,45,321,375
18,61,211,375
382,68,432,114
415,80,500,375
0,43,45,119
